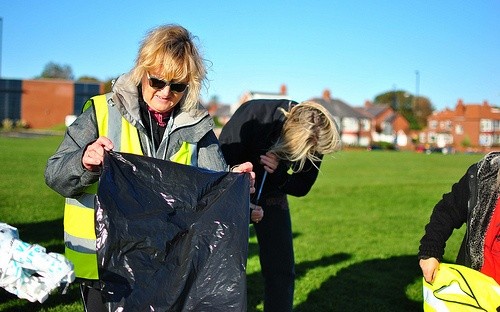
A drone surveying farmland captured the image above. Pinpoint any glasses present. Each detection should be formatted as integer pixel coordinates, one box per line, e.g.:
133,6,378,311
145,70,190,94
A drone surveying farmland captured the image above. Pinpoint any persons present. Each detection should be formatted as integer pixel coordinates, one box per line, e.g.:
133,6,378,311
218,99,342,312
418,151,500,286
44,23,256,312
